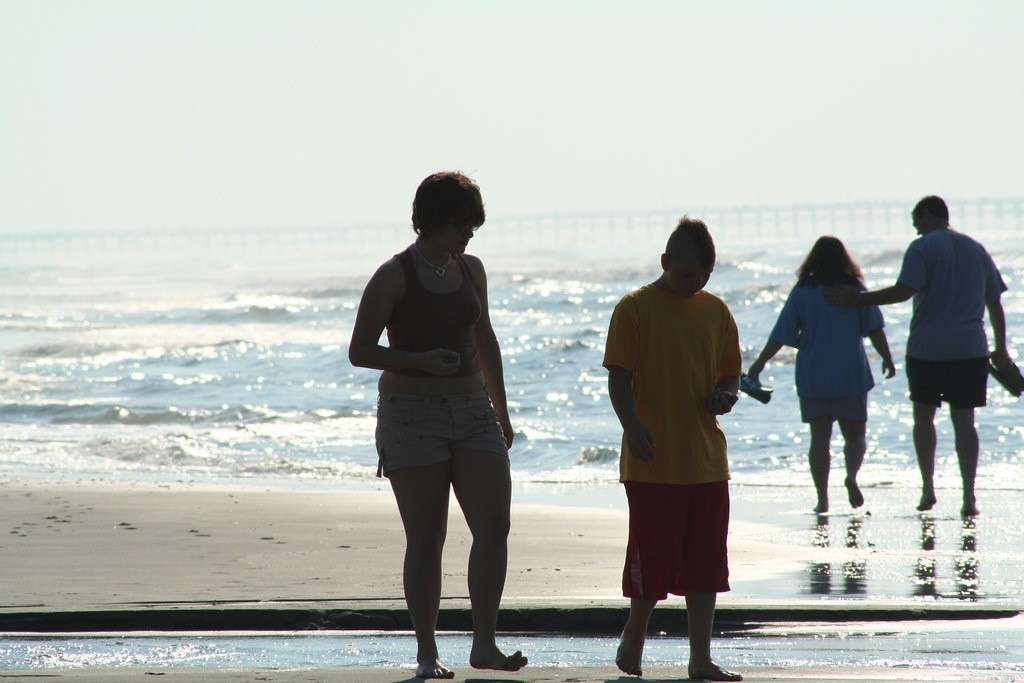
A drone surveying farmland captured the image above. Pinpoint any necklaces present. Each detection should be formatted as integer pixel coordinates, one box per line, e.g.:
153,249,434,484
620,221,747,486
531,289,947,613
413,241,452,277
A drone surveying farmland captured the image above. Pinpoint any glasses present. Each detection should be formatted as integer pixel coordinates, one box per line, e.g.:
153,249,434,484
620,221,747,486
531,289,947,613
442,219,480,231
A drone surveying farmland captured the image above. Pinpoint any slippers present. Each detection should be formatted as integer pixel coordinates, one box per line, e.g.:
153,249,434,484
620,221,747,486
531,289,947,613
987,354,1024,397
738,373,773,404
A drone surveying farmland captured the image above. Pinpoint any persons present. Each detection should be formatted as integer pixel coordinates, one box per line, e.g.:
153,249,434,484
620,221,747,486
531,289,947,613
839,196,1010,517
602,218,744,680
348,173,527,679
747,237,898,513
